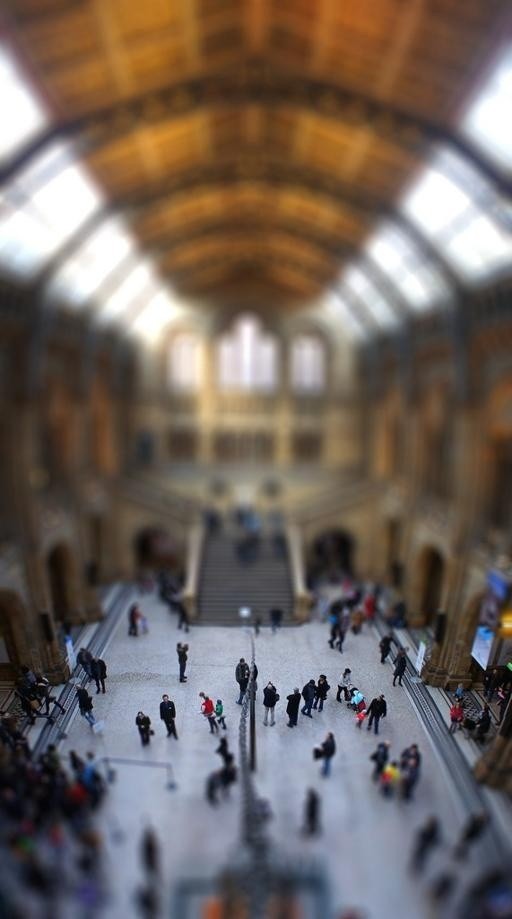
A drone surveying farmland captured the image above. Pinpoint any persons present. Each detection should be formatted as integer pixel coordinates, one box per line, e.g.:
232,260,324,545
1,507,508,919
